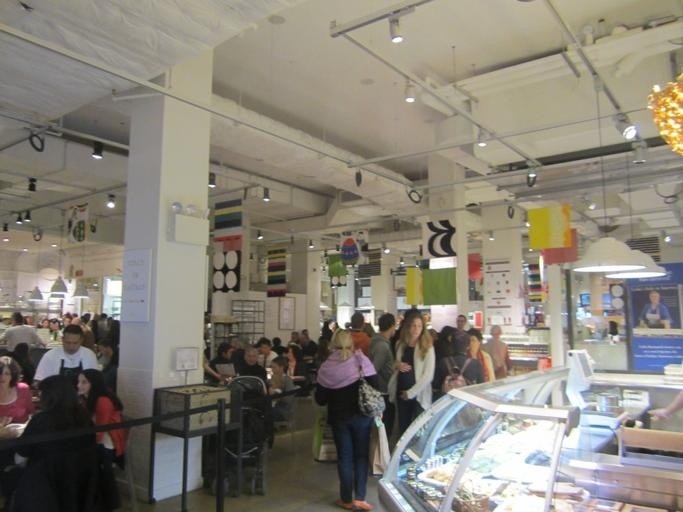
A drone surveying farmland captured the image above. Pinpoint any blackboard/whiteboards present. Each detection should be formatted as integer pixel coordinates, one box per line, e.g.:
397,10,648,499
278,296,296,330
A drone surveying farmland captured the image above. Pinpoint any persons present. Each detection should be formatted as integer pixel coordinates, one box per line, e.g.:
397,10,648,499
648,387,683,425
639,289,672,330
204,308,510,511
0,312,126,511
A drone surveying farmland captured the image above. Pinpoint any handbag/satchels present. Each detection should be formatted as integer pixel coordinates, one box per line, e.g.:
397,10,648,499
368,418,392,479
311,408,338,463
356,377,385,419
441,356,474,397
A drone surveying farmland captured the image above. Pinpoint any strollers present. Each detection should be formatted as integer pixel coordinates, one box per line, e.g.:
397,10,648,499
209,375,275,497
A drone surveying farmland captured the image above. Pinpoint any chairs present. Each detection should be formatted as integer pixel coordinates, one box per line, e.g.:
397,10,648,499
0,341,137,512
205,342,327,498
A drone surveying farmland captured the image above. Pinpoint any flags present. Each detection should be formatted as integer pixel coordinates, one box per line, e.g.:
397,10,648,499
531,202,576,251
61,204,91,247
545,228,580,267
211,195,242,294
338,226,374,269
266,246,288,299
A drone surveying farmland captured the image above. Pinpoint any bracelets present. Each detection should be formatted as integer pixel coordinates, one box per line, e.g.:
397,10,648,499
661,409,675,420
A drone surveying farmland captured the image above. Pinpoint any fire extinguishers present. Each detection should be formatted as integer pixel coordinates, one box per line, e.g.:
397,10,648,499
203,310,212,361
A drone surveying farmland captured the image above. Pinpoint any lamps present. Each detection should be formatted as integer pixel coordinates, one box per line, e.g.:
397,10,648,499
205,169,421,291
88,141,103,161
25,208,89,302
0,187,119,244
614,115,636,144
569,78,669,283
386,17,543,190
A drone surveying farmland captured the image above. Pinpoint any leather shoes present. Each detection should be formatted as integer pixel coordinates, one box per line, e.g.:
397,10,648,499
335,498,352,509
352,500,375,511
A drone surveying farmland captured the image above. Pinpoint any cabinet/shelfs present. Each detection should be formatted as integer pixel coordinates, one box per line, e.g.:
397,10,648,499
150,385,239,511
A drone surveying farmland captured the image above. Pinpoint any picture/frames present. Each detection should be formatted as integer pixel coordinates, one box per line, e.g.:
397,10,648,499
277,295,297,332
